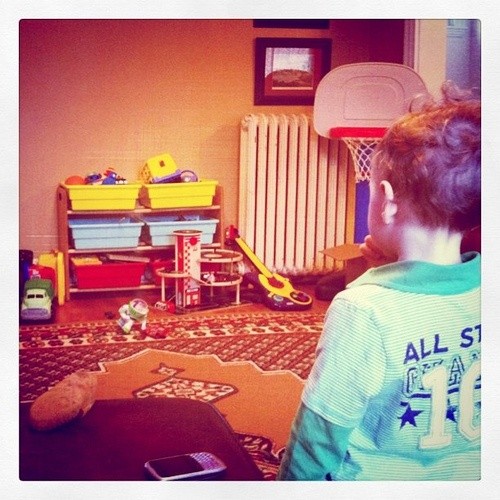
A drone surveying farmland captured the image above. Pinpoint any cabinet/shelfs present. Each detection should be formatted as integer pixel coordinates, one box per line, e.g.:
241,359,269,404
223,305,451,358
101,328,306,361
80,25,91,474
56,183,224,302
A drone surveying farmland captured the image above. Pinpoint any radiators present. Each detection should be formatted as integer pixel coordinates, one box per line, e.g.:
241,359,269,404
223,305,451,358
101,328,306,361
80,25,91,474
237,113,356,275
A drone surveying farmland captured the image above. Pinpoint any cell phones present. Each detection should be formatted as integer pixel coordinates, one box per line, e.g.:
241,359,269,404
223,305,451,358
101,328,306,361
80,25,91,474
144,451,227,481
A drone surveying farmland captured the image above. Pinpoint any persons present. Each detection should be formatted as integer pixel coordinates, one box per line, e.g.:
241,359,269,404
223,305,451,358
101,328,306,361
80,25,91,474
278,79,481,480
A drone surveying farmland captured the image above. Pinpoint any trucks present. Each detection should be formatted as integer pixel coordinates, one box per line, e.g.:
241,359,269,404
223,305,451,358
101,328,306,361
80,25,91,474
21,279,56,324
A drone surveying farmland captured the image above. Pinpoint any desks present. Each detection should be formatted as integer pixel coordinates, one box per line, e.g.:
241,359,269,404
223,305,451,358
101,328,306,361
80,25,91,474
19,398,264,481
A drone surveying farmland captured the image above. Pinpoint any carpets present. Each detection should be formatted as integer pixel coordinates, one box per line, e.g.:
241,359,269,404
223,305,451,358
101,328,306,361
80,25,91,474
19,310,326,481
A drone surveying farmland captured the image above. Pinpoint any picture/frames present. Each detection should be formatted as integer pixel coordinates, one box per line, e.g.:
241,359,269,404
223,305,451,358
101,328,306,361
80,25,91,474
253,37,332,106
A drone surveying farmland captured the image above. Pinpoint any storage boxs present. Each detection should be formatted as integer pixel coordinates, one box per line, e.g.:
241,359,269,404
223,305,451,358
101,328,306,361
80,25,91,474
137,214,220,246
320,243,368,286
135,178,220,209
70,255,146,289
139,251,221,286
60,181,142,211
68,217,145,249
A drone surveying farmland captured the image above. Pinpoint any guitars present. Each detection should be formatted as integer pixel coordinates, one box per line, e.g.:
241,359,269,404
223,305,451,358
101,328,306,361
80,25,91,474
224,224,313,311
